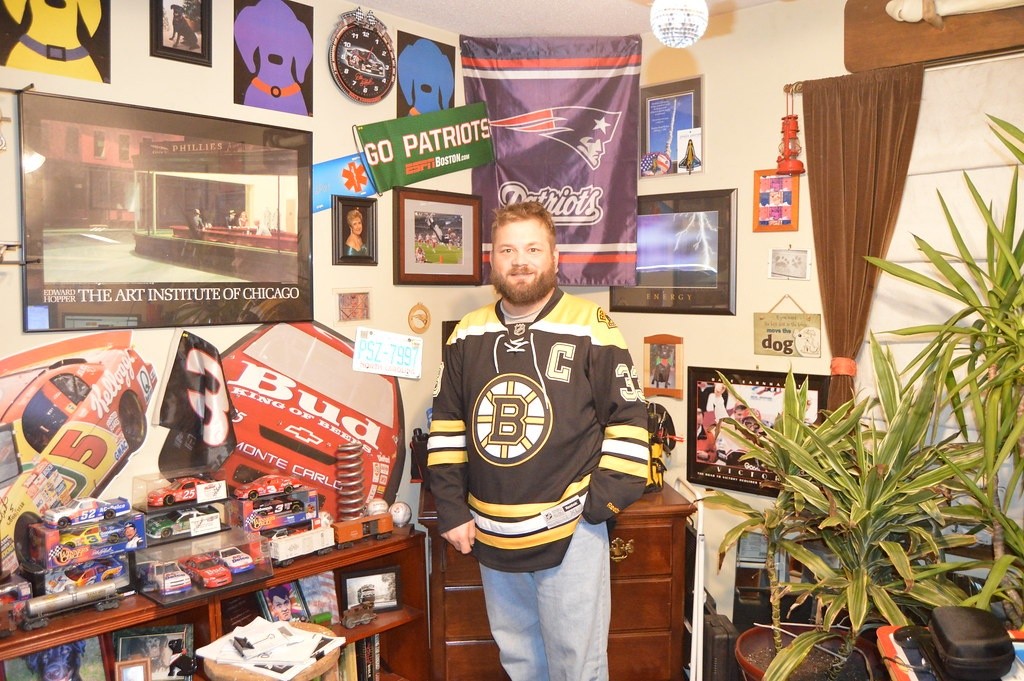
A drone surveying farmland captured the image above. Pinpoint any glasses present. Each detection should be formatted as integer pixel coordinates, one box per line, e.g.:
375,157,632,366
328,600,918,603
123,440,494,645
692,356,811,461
743,421,759,429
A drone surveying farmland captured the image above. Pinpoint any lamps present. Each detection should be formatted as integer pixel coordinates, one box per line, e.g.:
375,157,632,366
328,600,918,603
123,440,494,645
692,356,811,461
651,0,709,49
776,116,805,174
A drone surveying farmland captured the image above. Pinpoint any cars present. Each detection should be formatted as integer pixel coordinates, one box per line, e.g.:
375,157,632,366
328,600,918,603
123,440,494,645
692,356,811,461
0,330,157,582
234,474,302,500
253,496,304,516
45,555,126,594
206,547,254,573
177,555,232,588
59,521,125,549
145,505,220,538
137,561,191,596
43,496,132,529
147,477,217,507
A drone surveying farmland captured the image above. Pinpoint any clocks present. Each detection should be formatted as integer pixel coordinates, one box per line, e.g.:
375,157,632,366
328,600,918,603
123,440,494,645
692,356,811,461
329,7,397,105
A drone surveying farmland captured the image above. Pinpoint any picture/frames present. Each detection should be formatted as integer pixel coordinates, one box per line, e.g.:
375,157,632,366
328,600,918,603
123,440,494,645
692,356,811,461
331,194,378,266
753,169,799,232
150,0,212,66
255,582,310,625
643,335,684,399
332,288,374,327
113,657,151,681
393,186,483,287
339,565,403,614
686,367,831,498
609,188,738,315
0,634,111,681
112,624,193,681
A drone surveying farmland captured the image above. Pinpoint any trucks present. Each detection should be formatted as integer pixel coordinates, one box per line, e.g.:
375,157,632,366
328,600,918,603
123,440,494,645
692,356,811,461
357,585,375,608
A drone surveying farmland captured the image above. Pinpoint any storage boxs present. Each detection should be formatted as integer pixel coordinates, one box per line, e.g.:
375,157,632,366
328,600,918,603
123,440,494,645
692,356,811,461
27,467,318,609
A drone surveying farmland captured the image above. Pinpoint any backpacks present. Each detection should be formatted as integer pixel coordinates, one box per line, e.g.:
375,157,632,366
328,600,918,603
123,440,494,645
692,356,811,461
642,401,678,494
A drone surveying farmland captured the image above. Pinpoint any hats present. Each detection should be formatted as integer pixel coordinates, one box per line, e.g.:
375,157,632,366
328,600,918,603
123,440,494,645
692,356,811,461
741,408,761,425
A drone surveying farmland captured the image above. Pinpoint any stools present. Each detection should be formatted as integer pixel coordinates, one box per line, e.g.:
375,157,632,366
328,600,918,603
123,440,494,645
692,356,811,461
203,623,339,681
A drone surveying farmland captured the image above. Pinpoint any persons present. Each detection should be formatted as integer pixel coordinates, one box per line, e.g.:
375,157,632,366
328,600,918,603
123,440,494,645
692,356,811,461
415,232,462,264
268,585,299,623
425,203,650,681
697,383,766,470
343,211,368,257
188,209,272,241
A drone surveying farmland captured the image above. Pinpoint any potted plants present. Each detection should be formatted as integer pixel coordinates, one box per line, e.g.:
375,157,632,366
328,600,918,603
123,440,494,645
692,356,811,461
692,114,1024,681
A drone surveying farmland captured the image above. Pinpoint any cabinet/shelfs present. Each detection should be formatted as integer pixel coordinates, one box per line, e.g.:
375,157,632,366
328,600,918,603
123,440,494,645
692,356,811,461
1,522,429,681
424,515,685,681
